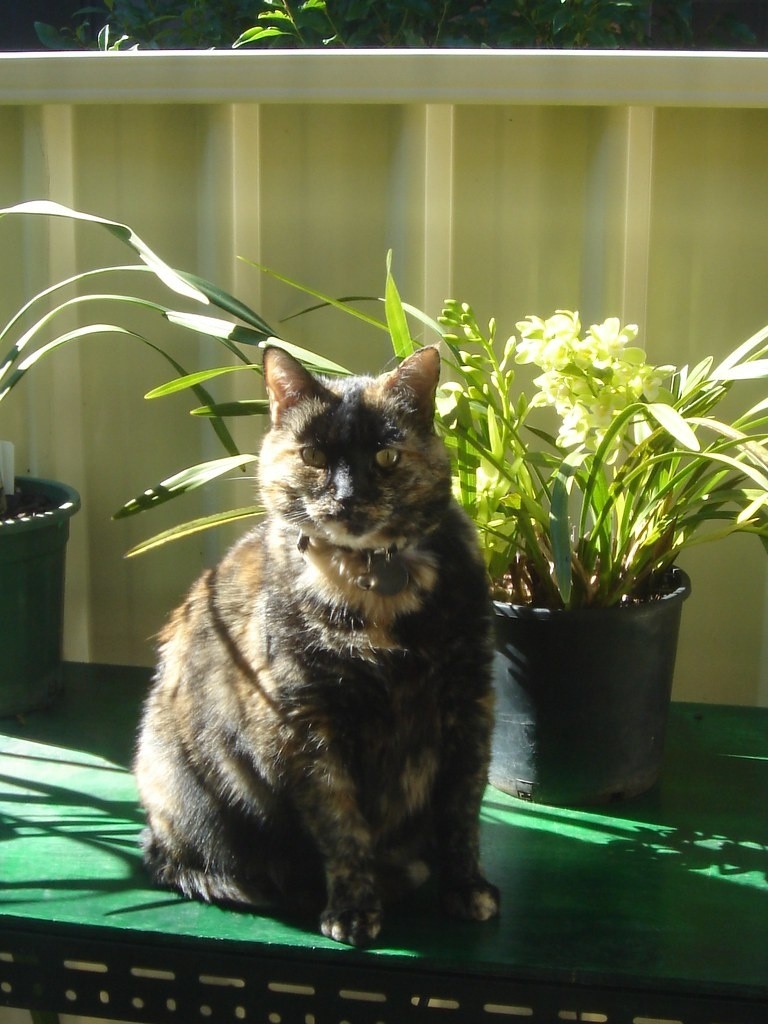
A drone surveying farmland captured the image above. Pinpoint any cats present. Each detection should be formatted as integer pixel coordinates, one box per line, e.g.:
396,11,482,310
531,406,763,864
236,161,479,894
136,346,500,948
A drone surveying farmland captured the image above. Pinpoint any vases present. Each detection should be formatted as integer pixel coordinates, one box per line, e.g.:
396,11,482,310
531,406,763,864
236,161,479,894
489,564,691,805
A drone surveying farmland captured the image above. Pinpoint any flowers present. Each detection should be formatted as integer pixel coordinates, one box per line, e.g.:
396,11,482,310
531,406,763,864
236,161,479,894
110,249,768,608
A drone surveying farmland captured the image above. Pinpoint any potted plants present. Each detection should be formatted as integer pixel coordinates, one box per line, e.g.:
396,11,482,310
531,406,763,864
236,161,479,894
0,200,285,719
